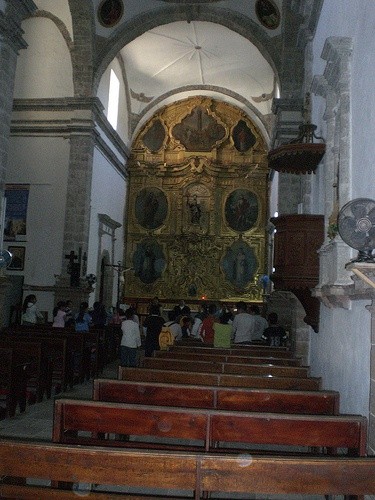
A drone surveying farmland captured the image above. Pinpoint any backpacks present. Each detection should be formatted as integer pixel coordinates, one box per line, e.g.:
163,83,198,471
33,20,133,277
159,322,176,350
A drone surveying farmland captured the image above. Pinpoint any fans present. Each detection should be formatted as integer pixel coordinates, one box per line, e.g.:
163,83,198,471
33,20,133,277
337,198,375,263
0,249,12,277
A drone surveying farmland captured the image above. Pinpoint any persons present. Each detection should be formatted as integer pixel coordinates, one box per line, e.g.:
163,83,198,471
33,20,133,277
142,306,166,357
232,301,256,344
21,294,44,326
64,300,73,324
177,307,194,340
174,299,191,319
162,311,183,341
259,313,288,346
147,297,163,316
190,301,235,348
88,301,107,333
52,301,67,328
120,309,142,368
75,302,92,332
249,305,269,341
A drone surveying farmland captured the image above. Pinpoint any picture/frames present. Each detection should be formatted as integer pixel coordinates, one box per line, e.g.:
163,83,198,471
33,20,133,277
7,246,26,271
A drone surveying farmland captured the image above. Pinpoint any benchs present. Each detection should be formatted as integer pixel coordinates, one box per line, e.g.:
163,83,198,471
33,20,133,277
1,321,374,500
